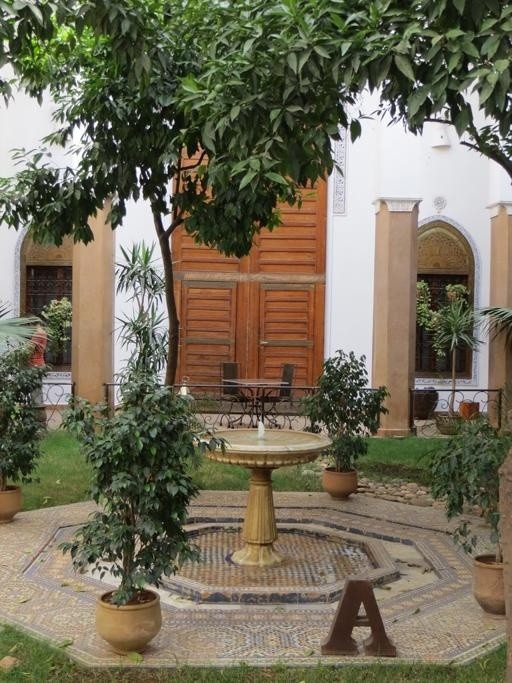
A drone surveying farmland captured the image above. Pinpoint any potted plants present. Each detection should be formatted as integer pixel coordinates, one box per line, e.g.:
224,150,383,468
296,348,388,500
57,379,231,655
428,298,486,435
0,346,53,522
410,427,512,614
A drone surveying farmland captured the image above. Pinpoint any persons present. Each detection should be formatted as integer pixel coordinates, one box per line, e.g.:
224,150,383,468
22,313,47,407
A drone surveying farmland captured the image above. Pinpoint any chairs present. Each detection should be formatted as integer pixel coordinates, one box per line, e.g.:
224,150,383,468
219,362,297,428
